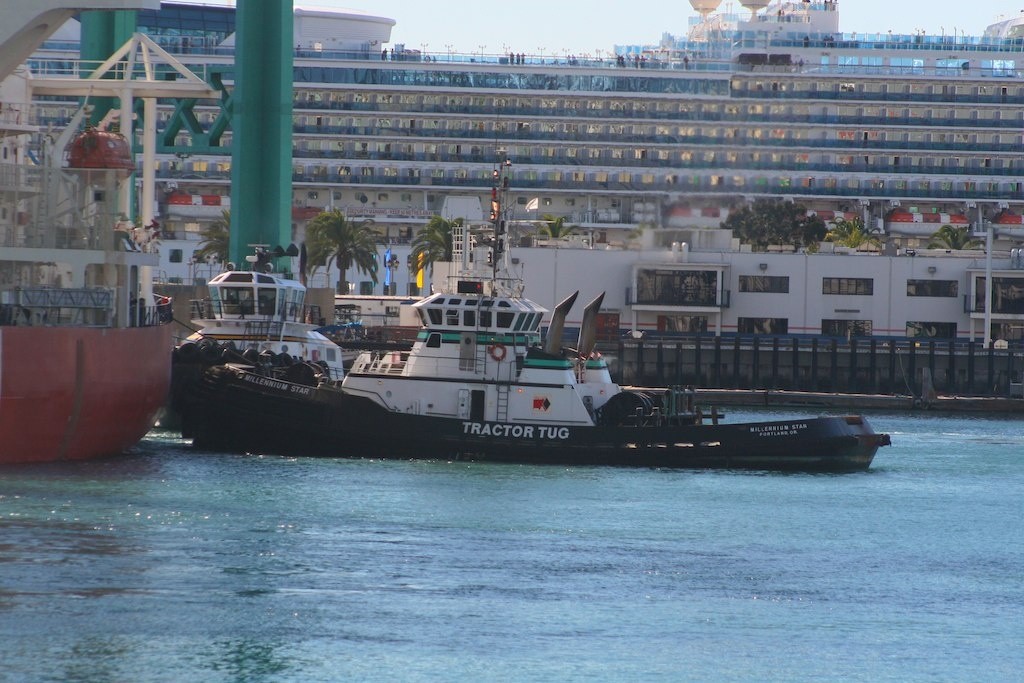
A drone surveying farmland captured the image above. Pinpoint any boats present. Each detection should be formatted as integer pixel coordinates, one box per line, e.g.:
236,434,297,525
665,204,737,230
992,211,1024,243
886,206,970,240
65,130,138,171
193,147,892,474
166,189,230,220
170,240,346,439
795,208,861,233
1,0,225,467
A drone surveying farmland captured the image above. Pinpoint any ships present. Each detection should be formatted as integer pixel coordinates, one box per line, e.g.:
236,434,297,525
25,0,1024,298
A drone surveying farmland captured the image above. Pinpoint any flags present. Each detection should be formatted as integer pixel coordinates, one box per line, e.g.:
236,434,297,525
385,248,391,285
525,198,538,212
417,250,423,287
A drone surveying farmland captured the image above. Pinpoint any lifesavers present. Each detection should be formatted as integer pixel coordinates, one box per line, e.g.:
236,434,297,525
489,343,506,362
171,342,330,378
16,113,22,126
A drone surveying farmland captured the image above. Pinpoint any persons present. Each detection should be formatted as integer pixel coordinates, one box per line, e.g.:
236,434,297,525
296,45,301,57
635,54,648,68
509,52,526,65
616,55,624,67
382,48,395,61
683,55,689,70
824,35,834,48
